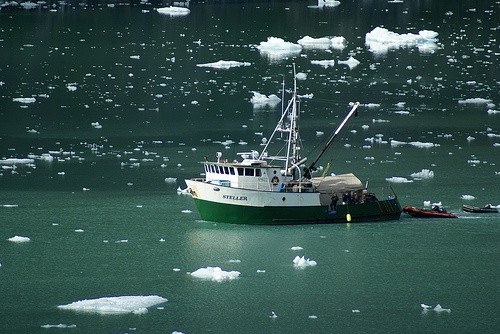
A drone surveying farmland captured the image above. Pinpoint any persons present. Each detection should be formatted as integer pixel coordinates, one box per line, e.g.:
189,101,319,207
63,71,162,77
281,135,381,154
330,192,339,213
342,192,358,205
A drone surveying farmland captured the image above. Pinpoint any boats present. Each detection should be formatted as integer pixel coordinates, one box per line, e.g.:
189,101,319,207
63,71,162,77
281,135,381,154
184,61,404,226
402,205,458,218
462,205,500,213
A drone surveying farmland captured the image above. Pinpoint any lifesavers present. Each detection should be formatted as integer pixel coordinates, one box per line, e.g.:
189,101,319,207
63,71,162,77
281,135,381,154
291,183,299,192
271,176,279,186
